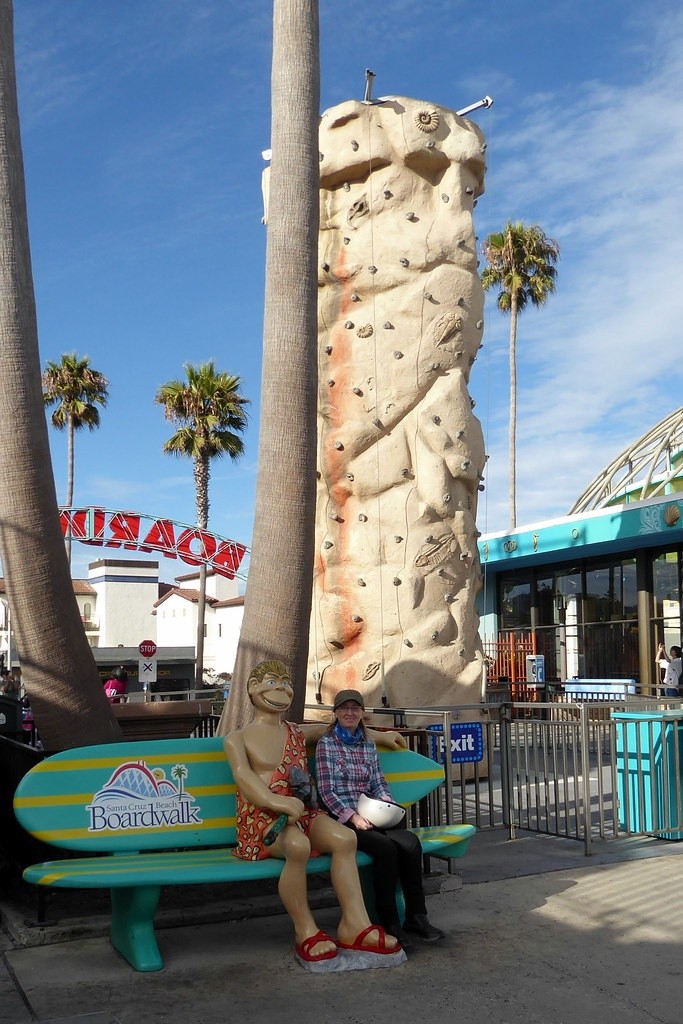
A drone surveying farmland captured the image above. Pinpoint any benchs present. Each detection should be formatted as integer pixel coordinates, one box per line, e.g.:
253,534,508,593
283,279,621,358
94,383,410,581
9,736,480,973
566,678,636,701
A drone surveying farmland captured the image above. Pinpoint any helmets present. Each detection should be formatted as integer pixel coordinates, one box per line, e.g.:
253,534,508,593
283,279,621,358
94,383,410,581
356,792,405,829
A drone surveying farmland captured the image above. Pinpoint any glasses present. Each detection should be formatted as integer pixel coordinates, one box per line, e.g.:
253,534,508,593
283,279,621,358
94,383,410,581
337,704,363,713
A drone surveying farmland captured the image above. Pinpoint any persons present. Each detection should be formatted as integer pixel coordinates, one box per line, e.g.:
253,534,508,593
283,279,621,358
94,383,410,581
317,688,447,943
227,660,407,959
103,667,129,705
654,643,682,697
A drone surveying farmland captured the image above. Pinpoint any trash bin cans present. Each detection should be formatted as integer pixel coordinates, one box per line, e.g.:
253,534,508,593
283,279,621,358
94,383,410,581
0,695,25,744
609,708,683,841
487,675,511,720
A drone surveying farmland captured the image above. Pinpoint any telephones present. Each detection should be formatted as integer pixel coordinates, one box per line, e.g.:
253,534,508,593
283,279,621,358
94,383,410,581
531,661,536,677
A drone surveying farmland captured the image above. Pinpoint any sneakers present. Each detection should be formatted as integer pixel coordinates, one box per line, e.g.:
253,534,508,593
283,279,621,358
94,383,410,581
380,921,416,947
402,911,444,942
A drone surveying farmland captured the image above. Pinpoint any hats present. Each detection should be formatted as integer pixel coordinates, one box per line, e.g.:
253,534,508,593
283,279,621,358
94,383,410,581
0,669,11,676
333,689,364,714
